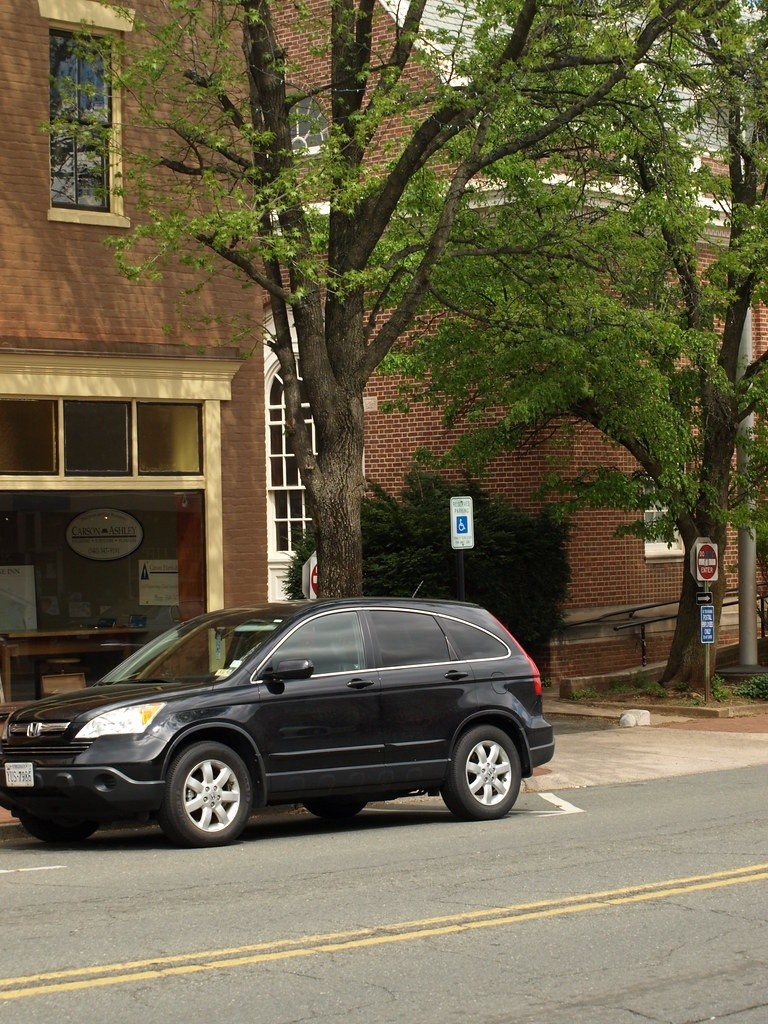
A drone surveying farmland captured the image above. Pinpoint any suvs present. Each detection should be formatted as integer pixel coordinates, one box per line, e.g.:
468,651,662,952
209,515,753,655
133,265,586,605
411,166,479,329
1,596,555,848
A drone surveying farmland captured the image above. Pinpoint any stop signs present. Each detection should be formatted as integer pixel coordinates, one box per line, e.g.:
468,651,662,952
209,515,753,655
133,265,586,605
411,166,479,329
697,544,718,579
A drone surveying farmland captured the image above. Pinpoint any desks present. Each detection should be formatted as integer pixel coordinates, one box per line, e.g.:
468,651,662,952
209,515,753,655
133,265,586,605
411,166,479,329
0,629,152,703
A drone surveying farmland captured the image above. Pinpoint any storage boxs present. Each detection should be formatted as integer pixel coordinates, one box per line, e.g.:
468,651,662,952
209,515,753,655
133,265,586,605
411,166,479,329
40,673,87,696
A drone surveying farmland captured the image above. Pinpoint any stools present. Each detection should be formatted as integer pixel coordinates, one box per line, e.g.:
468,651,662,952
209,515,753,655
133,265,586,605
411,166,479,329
47,658,79,674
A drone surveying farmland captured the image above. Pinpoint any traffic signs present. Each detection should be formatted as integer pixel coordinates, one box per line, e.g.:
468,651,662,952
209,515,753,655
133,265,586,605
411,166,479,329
696,591,715,642
